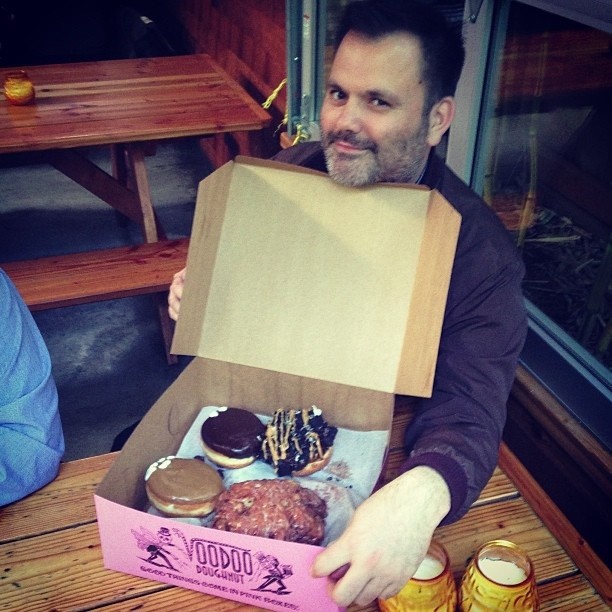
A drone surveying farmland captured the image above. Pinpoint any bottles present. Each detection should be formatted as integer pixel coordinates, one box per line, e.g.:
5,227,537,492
3,70,35,106
378,541,457,612
459,540,540,611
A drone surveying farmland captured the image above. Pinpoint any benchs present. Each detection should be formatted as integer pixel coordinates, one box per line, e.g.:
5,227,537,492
2,236,191,365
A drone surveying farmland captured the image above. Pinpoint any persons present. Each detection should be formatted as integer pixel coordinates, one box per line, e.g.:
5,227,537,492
0,263,65,511
109,0,530,607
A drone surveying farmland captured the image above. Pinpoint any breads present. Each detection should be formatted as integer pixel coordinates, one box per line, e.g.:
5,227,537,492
144,455,224,516
264,407,339,478
201,407,265,468
213,480,326,545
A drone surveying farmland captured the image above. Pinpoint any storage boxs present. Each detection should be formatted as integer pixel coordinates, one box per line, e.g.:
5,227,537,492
92,155,462,612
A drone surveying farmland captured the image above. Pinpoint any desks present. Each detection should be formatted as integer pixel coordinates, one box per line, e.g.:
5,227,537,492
1,398,611,612
0,52,272,245
